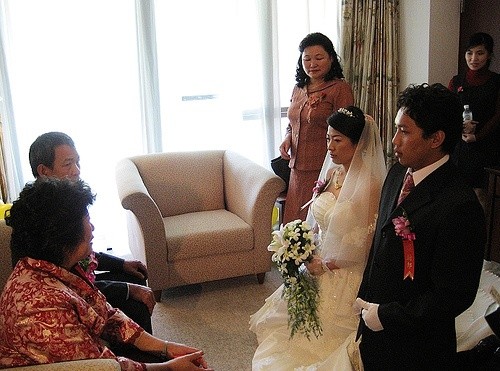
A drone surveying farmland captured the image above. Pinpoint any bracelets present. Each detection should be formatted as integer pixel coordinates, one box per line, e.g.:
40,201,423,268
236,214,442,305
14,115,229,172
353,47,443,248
162,340,170,357
321,260,331,273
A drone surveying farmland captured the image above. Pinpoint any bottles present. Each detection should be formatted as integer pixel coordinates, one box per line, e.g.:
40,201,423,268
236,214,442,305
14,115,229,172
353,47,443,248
462,103,475,123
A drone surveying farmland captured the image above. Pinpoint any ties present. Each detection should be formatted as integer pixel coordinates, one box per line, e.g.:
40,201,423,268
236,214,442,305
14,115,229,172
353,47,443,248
395,171,415,208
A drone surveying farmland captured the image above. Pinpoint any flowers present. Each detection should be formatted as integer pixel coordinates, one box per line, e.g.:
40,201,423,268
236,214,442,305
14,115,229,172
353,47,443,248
312,180,326,193
267,219,324,275
306,94,322,109
392,215,417,241
74,253,99,283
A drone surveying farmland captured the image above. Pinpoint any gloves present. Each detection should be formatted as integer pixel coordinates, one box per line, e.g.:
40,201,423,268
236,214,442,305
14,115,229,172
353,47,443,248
355,297,384,332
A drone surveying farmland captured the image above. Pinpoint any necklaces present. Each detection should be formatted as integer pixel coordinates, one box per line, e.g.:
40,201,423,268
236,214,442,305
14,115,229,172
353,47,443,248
335,167,343,191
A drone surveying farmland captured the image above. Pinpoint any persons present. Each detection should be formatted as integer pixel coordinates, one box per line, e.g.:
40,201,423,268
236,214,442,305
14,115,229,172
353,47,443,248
11,132,152,364
248,106,387,371
448,32,500,173
0,175,215,371
279,32,355,226
353,83,489,371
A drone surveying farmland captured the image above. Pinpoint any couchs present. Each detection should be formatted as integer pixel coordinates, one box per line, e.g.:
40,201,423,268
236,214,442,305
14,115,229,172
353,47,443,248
116,150,286,302
